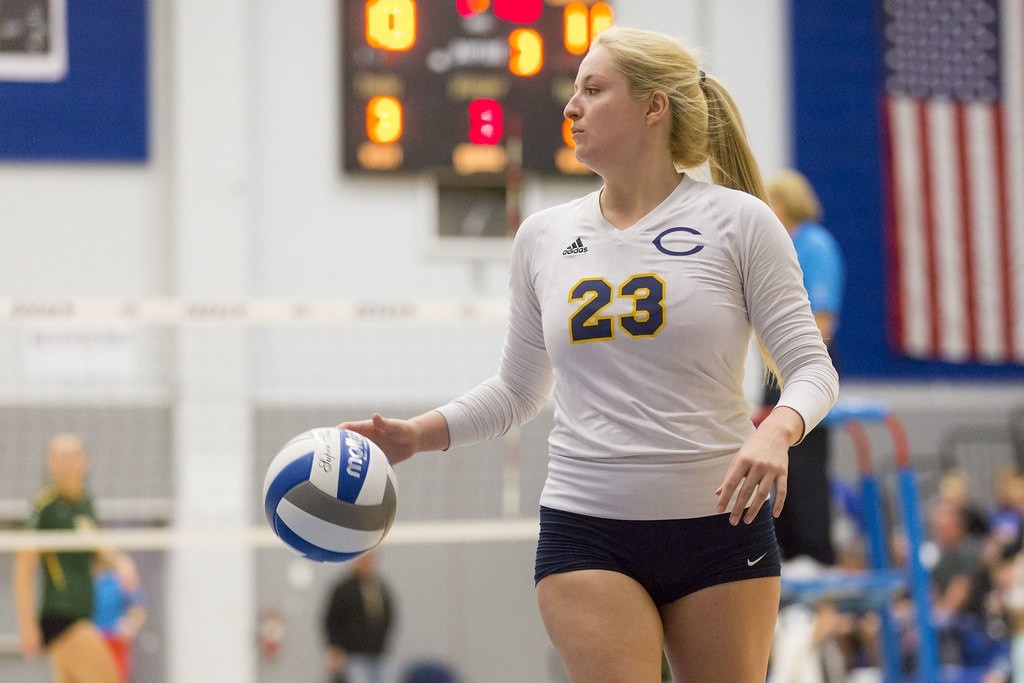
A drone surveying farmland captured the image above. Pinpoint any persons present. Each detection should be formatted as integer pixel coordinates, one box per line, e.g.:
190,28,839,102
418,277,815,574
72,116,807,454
338,26,843,682
86,558,147,682
14,437,140,682
803,459,1023,683
322,547,394,682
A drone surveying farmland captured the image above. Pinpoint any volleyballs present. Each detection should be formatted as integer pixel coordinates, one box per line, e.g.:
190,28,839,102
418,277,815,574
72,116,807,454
261,427,397,562
